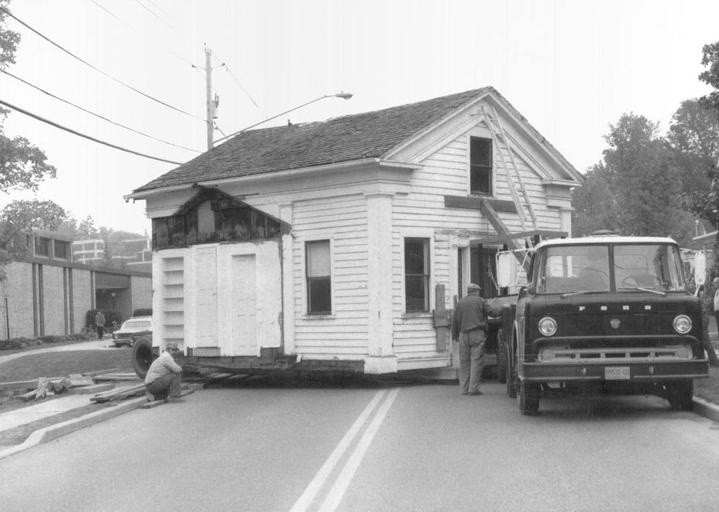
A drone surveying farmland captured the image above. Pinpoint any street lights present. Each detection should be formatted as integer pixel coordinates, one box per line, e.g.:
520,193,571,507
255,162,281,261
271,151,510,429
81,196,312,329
206,88,355,150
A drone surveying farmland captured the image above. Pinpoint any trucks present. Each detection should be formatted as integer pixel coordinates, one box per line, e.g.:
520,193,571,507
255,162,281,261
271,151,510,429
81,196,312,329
493,233,710,416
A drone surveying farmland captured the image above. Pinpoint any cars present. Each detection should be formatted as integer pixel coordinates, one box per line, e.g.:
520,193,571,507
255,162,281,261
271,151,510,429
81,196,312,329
111,315,154,347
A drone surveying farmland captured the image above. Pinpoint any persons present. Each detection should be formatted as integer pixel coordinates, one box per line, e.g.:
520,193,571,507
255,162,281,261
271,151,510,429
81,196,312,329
712,277,719,330
95,310,105,340
452,283,511,396
144,342,185,402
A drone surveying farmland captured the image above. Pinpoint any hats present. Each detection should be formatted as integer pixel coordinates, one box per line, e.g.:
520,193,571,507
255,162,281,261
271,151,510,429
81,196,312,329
166,342,179,351
468,282,482,291
